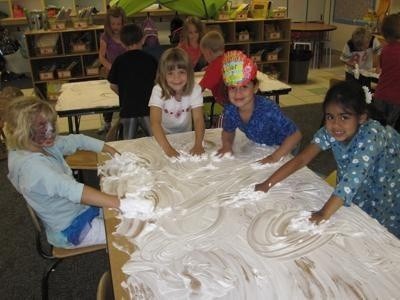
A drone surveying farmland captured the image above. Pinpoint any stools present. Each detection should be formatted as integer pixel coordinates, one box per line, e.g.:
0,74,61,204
63,150,97,186
292,40,312,52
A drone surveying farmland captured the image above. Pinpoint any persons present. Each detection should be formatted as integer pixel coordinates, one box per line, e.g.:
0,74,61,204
148,47,206,159
95,6,128,135
107,23,158,140
0,96,153,249
176,15,203,70
371,14,400,128
339,27,384,80
197,31,231,129
254,76,400,226
214,50,302,165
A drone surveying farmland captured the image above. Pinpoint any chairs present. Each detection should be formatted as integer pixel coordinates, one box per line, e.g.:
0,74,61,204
314,30,334,69
96,270,113,300
23,201,108,300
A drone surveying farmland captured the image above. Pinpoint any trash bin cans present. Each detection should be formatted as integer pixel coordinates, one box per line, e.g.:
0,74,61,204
289,49,314,85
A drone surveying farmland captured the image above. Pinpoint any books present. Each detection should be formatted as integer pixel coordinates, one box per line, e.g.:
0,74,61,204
224,1,232,15
267,44,284,53
78,5,95,18
250,0,269,18
56,6,72,17
230,3,250,18
251,46,265,55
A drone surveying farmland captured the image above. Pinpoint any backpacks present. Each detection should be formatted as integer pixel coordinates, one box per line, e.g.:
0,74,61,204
142,17,160,48
169,14,183,44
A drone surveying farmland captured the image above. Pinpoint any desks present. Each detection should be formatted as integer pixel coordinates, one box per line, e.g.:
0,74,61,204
193,70,292,128
291,22,338,72
96,126,400,300
54,69,293,134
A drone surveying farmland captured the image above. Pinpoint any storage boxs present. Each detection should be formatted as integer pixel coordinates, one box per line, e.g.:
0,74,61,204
38,71,55,81
70,43,86,53
57,70,71,79
237,31,250,42
71,18,90,29
266,51,279,59
271,9,286,18
85,65,100,76
251,54,263,62
37,46,57,56
236,12,249,20
216,11,231,20
264,31,281,41
53,19,68,30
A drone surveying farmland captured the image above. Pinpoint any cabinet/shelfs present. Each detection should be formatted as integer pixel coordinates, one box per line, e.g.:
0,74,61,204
0,0,177,24
201,16,292,85
23,24,106,94
